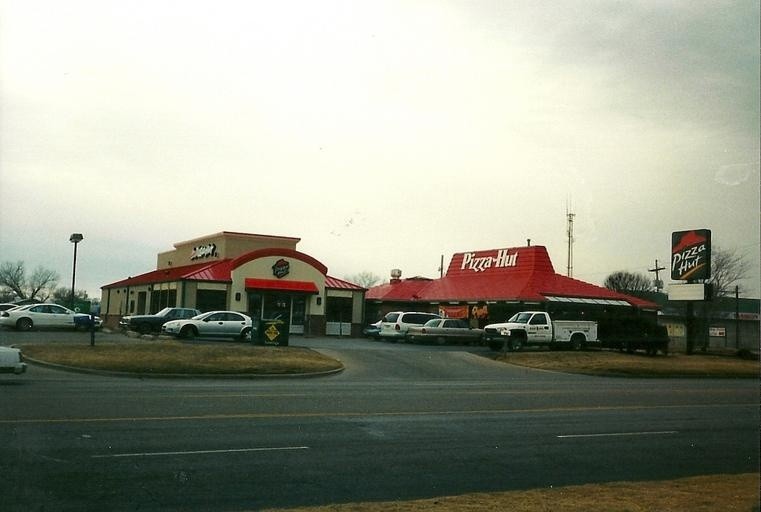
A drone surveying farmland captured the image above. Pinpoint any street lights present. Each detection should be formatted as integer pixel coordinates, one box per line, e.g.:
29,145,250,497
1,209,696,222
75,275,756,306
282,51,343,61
70,234,83,307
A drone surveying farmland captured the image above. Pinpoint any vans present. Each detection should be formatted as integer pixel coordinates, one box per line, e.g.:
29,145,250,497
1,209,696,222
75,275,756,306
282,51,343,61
379,312,443,339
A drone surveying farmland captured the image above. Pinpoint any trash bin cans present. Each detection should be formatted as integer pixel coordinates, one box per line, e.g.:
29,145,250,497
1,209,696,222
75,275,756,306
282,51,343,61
351,324,362,337
261,321,289,345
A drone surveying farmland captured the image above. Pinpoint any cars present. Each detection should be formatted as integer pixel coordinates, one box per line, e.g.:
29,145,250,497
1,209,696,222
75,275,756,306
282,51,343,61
0,303,104,331
407,319,486,345
120,307,203,333
363,319,383,337
161,310,252,343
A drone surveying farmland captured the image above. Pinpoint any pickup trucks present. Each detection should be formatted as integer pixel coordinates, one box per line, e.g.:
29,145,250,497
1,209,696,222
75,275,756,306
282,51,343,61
484,311,601,351
